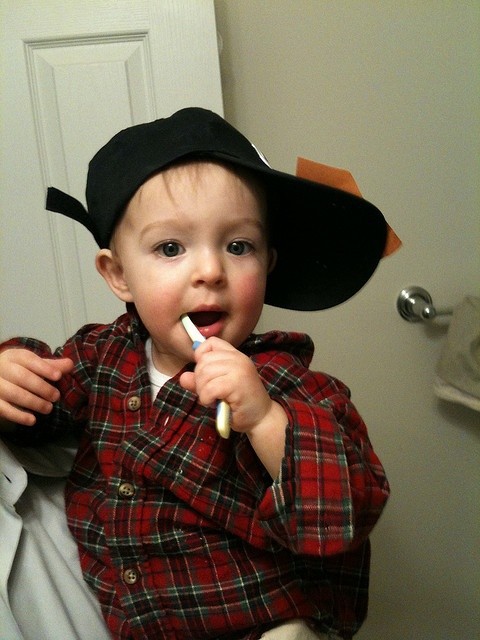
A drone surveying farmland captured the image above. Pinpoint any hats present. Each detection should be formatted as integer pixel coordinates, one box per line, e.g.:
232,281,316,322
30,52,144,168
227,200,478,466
45,107,385,312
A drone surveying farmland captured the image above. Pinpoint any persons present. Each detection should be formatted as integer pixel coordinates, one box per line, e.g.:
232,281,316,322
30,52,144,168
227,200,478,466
0,108,391,639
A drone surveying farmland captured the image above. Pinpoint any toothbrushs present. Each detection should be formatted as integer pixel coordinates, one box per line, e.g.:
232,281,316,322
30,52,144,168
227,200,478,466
171,310,237,444
